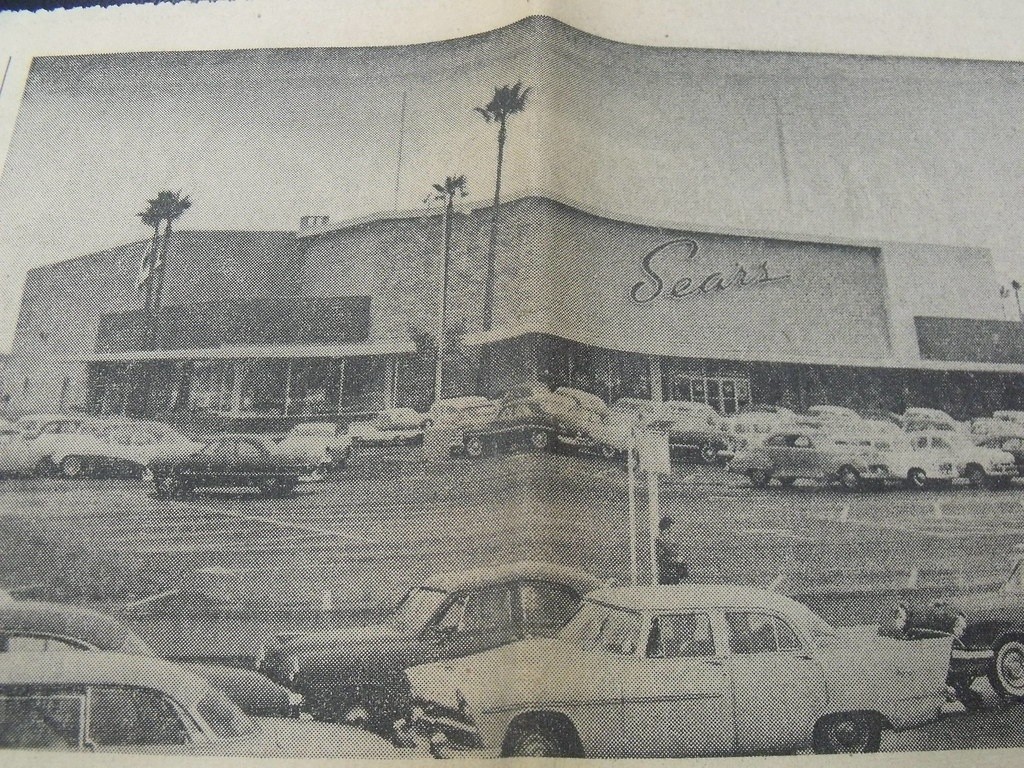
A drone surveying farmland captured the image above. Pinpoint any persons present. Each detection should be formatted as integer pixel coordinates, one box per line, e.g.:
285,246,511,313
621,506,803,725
652,518,688,584
661,615,699,656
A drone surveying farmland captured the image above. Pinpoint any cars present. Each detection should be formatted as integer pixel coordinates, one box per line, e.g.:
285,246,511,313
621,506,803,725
877,556,1023,703
392,582,952,760
277,566,611,738
0,388,1024,502
2,599,304,725
0,647,391,759
